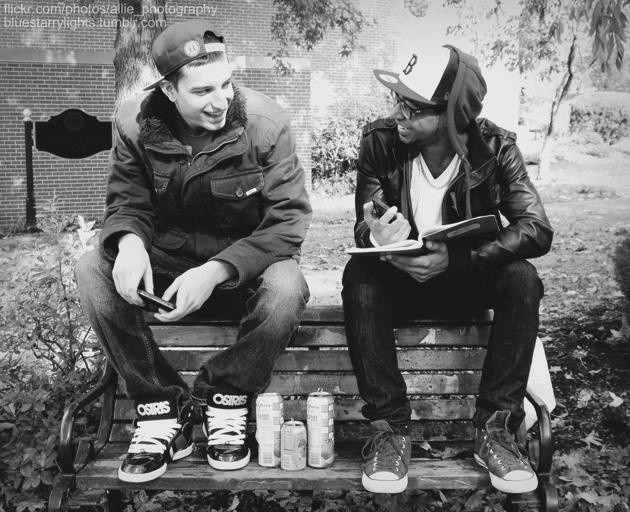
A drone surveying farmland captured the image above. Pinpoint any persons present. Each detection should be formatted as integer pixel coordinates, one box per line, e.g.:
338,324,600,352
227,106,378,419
341,44,554,493
73,20,313,482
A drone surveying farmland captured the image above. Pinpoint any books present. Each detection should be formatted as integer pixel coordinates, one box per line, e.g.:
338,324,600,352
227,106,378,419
347,214,501,260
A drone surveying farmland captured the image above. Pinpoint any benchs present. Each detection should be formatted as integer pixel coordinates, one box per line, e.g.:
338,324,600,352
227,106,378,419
47,301,558,512
523,138,547,178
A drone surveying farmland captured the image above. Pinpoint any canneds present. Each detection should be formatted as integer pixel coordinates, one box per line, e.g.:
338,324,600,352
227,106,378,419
306,387,335,469
280,421,307,471
254,392,284,467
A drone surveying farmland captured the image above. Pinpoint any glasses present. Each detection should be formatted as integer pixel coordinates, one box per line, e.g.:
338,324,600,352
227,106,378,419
391,90,445,119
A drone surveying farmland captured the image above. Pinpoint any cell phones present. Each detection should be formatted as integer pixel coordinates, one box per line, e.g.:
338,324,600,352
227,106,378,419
138,290,175,313
372,197,397,224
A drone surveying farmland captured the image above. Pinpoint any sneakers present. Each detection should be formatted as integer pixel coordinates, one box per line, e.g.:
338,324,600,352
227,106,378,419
474,410,538,494
362,417,410,494
116,391,194,484
201,388,250,471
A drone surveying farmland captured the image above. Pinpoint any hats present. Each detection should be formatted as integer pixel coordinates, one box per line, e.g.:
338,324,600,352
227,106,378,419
373,45,460,102
142,19,225,91
